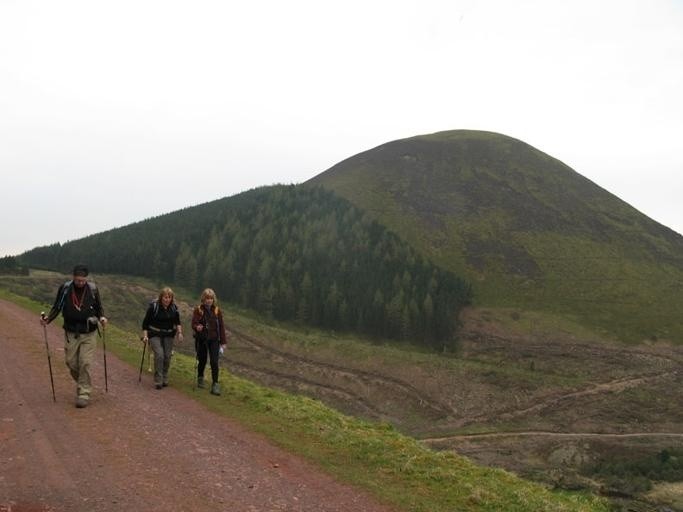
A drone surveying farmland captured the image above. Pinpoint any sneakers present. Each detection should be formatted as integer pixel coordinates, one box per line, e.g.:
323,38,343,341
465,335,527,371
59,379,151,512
74,397,88,408
210,382,220,396
154,383,169,391
197,376,205,388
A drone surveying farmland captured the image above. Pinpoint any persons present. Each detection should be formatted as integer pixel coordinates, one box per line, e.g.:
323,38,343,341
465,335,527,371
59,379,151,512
142,287,184,389
191,289,226,396
40,264,107,407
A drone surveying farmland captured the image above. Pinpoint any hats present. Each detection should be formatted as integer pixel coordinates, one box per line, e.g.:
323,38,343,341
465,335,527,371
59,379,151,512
72,264,89,277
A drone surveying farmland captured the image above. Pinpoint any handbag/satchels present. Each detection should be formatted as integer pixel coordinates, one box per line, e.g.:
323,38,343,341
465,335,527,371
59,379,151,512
74,309,104,339
